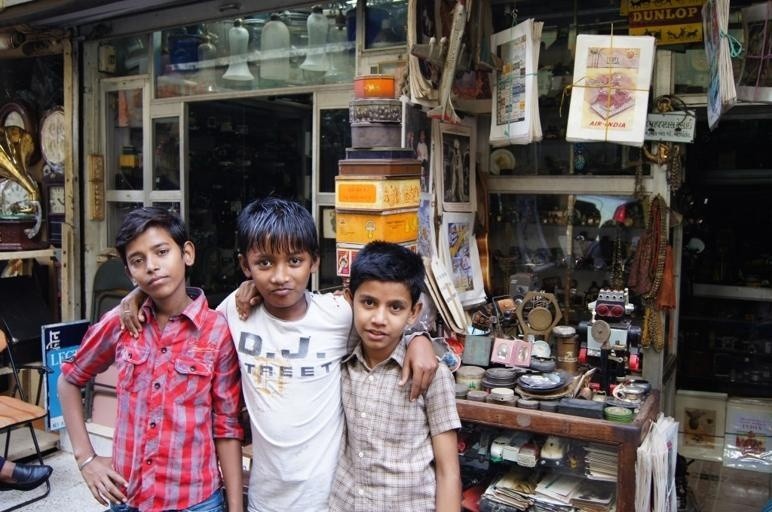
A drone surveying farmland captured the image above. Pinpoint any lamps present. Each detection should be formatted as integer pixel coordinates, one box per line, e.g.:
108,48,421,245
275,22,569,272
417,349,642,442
222,4,331,81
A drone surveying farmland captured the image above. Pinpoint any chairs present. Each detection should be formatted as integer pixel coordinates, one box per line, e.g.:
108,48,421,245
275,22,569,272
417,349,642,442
0,329,55,512
85,258,138,422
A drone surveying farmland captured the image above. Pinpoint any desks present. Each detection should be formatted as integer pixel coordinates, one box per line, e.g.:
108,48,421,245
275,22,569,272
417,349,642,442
456,390,660,512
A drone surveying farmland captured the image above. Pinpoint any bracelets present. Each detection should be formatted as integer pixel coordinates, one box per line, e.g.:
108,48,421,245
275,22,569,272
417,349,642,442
78,453,97,470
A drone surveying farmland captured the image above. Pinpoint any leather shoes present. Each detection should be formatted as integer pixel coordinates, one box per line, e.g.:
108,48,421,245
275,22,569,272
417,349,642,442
0,462,53,490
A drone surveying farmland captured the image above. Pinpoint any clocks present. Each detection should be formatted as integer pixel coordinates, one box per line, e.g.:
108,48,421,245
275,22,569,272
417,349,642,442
41,180,65,219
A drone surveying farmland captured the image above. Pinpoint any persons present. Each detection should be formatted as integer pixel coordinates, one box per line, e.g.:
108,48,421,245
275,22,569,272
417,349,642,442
415,127,427,191
234,240,464,512
118,197,439,512
57,206,247,512
0,455,53,492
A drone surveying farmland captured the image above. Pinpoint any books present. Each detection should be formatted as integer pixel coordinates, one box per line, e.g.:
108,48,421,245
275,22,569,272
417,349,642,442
460,473,616,512
568,33,656,148
631,413,680,512
487,18,546,144
580,442,618,483
421,256,469,334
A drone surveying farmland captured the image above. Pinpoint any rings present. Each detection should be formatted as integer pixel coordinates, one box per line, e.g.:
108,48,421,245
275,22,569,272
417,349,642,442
124,310,130,315
